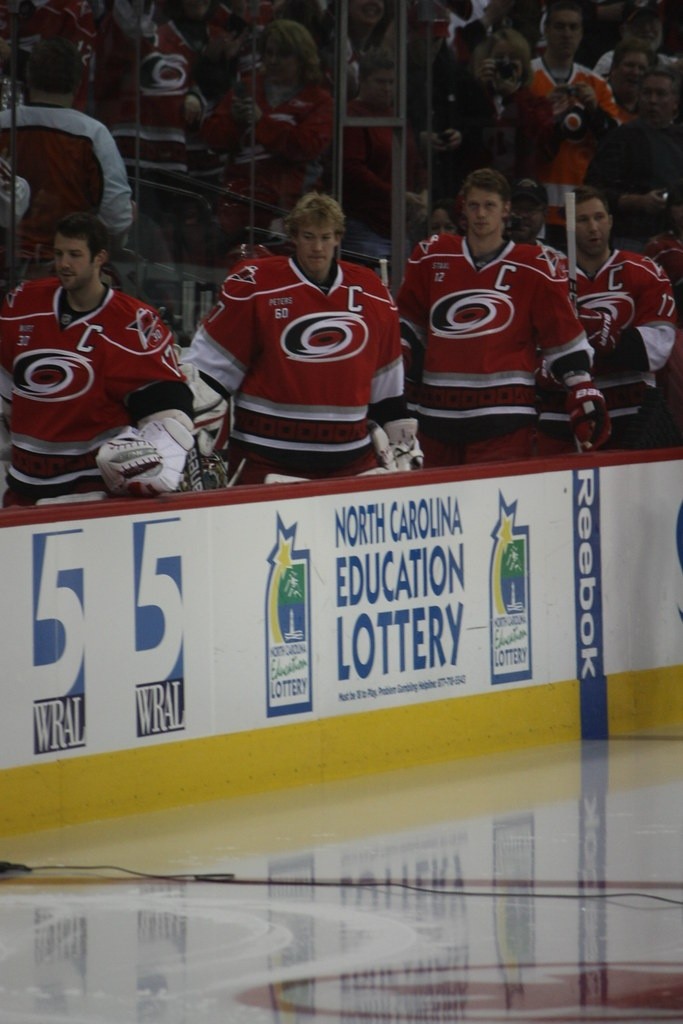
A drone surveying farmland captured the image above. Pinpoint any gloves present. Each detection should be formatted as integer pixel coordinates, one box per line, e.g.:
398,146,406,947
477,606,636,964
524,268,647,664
95,418,195,495
565,382,612,452
178,363,237,457
356,418,424,477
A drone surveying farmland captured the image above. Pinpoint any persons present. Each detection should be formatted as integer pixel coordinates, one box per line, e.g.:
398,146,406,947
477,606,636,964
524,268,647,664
0,211,194,508
394,168,611,469
0,2,683,290
533,186,676,450
642,186,683,451
183,192,424,489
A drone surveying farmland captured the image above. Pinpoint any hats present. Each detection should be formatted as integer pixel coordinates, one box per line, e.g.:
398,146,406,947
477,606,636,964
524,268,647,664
667,180,683,203
408,1,450,40
511,177,548,204
622,0,665,22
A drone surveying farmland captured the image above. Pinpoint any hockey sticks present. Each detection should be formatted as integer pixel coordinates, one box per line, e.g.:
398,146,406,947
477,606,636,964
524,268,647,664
169,342,206,493
378,256,390,288
564,191,579,321
